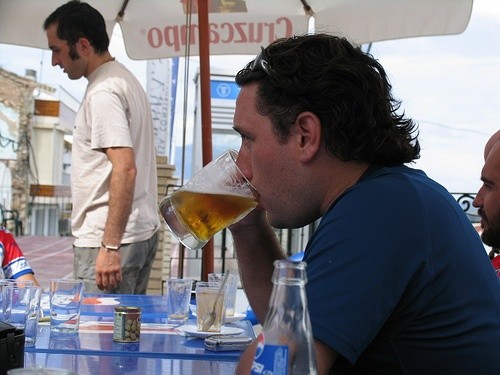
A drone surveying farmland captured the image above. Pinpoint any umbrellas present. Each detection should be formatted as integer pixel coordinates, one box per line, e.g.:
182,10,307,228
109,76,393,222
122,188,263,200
0,0,474,282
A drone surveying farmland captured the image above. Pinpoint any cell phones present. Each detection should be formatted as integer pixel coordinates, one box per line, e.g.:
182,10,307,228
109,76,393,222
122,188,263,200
204,336,253,351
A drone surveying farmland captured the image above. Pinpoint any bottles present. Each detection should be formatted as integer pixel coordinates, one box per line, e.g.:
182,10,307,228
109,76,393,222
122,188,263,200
250,260,318,375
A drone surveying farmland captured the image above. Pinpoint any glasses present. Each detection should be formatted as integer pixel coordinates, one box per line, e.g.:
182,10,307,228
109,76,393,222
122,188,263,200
249,49,280,84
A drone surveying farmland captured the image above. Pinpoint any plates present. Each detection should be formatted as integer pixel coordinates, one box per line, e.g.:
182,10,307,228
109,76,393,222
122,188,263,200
7,368,78,375
191,312,247,324
181,325,244,339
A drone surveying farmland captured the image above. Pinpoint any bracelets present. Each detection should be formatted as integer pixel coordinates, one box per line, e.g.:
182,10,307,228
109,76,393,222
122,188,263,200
102,242,121,251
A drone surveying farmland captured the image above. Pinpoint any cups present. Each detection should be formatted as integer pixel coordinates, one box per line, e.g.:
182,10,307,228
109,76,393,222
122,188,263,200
159,148,259,251
50,279,82,340
166,279,192,324
0,280,33,330
3,286,41,347
208,272,237,316
196,281,225,332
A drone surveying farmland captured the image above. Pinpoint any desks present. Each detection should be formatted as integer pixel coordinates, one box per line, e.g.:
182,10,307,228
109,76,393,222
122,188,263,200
24,294,257,375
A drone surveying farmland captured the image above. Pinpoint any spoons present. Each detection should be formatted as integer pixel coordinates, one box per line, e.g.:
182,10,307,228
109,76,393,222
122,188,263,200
202,269,230,331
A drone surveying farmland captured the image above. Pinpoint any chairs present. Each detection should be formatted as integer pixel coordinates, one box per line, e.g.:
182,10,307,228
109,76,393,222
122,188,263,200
0,203,23,237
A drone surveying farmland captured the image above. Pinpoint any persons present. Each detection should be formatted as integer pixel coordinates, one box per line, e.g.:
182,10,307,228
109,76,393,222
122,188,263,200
214,36,500,375
473,131,500,277
0,226,41,287
42,0,161,296
